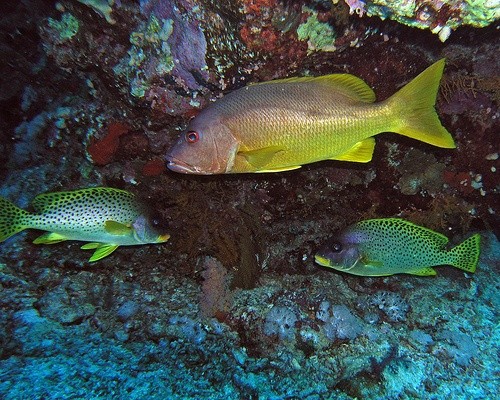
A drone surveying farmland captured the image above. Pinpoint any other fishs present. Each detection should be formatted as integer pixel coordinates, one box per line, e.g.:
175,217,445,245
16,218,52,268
0,187,171,262
315,218,481,276
164,57,456,175
